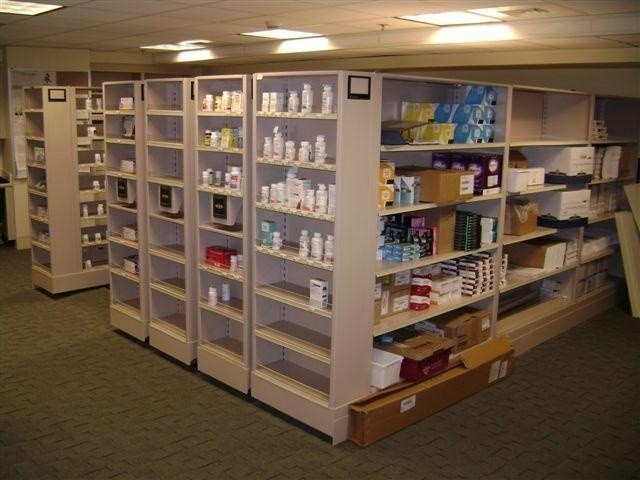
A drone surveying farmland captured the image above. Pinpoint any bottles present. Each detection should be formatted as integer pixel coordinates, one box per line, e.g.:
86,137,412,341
298,230,334,264
94,153,101,164
221,283,231,301
201,167,241,190
263,131,327,164
208,285,217,306
204,91,230,111
288,83,334,114
259,173,327,215
270,232,282,251
82,204,104,270
93,180,99,191
91,127,97,136
85,97,90,108
231,255,237,271
203,130,221,147
